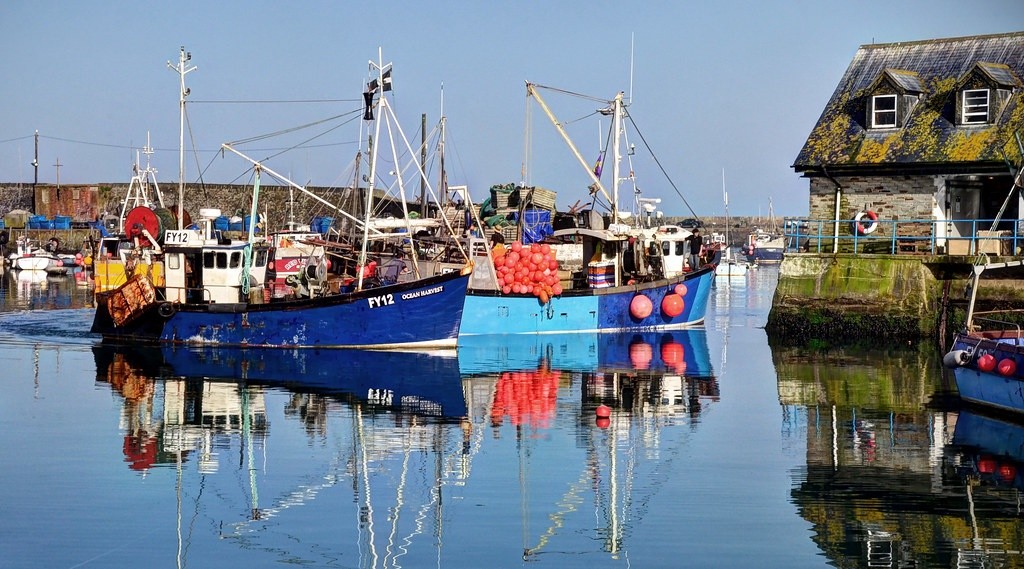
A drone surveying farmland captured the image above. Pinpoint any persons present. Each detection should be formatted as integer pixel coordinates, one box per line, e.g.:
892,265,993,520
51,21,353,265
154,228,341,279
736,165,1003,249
489,225,504,249
384,253,409,285
685,229,703,271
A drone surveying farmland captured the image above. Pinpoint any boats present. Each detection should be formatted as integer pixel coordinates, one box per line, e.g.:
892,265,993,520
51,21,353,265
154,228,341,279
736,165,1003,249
739,195,786,264
15,269,68,284
949,406,1024,555
0,27,727,348
454,320,722,562
89,343,474,568
949,160,1024,420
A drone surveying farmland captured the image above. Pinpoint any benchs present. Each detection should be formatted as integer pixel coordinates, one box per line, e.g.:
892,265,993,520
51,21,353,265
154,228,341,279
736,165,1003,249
897,223,932,255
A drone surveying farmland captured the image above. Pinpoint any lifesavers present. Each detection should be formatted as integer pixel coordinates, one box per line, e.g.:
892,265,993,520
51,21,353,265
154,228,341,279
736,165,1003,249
854,210,878,234
158,303,176,319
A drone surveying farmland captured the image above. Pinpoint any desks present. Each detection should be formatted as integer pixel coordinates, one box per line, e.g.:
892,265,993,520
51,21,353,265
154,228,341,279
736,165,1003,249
978,230,1012,256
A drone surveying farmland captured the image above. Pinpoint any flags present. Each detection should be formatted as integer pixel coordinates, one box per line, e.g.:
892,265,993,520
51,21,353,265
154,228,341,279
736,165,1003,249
592,155,602,178
367,68,392,93
